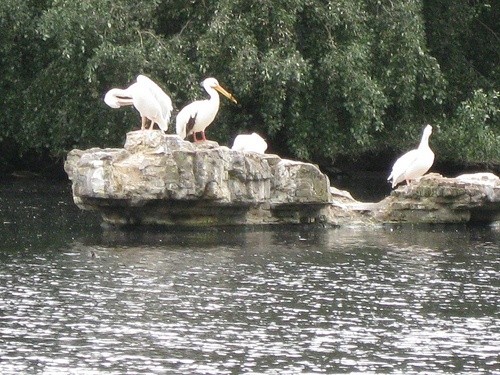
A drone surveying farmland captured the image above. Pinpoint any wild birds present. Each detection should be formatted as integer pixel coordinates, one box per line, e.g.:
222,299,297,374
386,123,436,190
231,132,268,155
102,74,174,132
175,77,237,144
